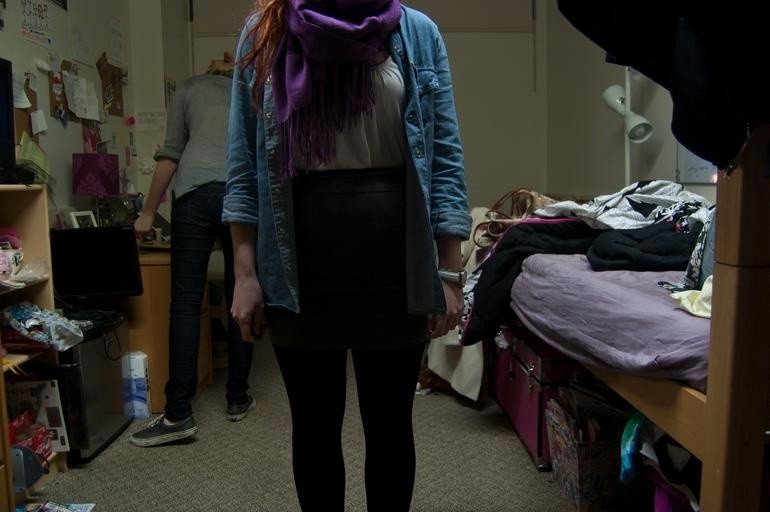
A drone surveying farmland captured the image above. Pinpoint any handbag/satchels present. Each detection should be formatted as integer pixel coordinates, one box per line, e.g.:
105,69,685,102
473,188,560,247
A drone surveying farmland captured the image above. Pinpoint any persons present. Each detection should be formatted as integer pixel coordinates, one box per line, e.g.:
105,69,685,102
221,0,483,511
122,49,257,449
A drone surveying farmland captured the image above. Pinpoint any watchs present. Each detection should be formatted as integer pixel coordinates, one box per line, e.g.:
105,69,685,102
435,268,470,288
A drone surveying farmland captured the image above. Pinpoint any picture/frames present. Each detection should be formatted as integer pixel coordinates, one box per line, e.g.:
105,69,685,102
69,209,98,230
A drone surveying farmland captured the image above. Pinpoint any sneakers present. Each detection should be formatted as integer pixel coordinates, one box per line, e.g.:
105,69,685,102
130,413,200,448
225,394,257,422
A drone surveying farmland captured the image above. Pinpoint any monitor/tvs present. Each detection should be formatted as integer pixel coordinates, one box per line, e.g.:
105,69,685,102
48,225,145,321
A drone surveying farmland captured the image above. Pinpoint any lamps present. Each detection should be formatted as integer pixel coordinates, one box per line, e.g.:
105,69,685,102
602,62,655,189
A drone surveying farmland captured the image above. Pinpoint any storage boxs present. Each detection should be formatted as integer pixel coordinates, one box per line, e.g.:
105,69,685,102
487,327,641,512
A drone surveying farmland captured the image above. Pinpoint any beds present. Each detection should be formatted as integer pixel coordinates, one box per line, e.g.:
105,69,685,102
504,131,770,512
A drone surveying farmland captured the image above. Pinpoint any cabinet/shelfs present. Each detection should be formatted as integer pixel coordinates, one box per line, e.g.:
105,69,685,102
0,182,71,510
55,308,135,468
123,250,216,417
